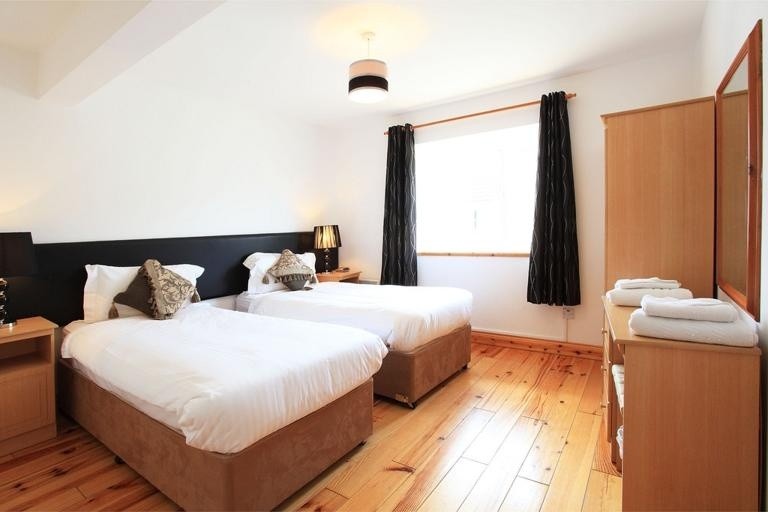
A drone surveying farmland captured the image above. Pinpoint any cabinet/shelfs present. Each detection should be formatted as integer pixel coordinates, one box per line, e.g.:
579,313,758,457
601,313,625,472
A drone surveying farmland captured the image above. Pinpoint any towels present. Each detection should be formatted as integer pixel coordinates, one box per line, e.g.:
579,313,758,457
628,309,759,348
614,277,681,291
641,295,737,323
606,288,693,306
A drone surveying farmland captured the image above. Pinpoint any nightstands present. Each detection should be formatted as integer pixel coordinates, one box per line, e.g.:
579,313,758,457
317,271,362,283
0,316,59,457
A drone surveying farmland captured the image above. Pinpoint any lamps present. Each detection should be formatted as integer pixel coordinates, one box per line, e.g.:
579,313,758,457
348,33,388,102
313,225,342,273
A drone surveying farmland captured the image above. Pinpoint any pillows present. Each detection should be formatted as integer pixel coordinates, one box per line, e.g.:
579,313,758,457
83,263,205,323
268,249,313,290
108,259,194,321
243,251,316,294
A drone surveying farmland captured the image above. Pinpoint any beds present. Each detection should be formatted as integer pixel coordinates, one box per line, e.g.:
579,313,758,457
235,282,472,405
62,299,389,512
